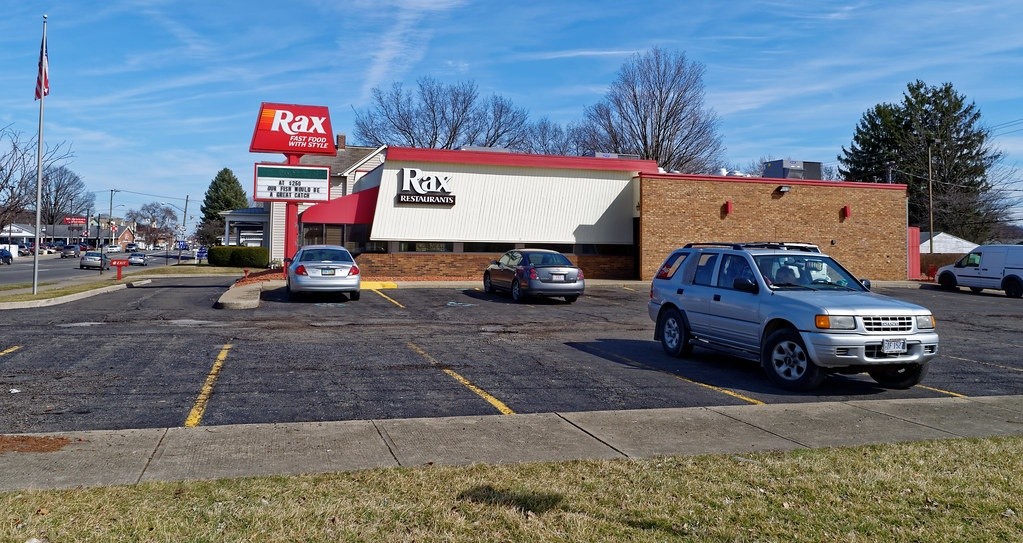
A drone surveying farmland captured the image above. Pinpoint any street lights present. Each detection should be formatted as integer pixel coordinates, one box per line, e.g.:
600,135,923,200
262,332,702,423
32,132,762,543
108,204,126,246
162,202,187,265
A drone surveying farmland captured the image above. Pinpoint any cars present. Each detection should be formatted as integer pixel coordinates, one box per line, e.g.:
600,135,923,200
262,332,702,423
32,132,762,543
153,246,161,250
483,248,585,303
77,242,94,250
284,245,362,302
107,245,121,253
60,245,80,258
125,243,139,252
80,252,111,272
128,253,149,266
719,240,834,284
0,249,14,265
13,241,66,257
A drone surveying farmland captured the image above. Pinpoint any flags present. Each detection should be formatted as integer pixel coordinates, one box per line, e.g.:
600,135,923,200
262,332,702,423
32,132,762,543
34,37,49,100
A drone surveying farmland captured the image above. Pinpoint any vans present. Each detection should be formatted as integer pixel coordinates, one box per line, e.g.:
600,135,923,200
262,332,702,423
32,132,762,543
933,244,1023,297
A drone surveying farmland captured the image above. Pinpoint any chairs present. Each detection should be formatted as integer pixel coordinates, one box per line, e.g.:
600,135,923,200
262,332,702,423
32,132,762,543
694,256,811,294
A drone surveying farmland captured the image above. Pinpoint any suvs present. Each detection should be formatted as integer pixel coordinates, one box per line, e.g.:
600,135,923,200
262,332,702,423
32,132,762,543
647,241,940,392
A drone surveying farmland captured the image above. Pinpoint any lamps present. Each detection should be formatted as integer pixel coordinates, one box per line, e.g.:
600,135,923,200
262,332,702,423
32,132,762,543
778,185,791,196
